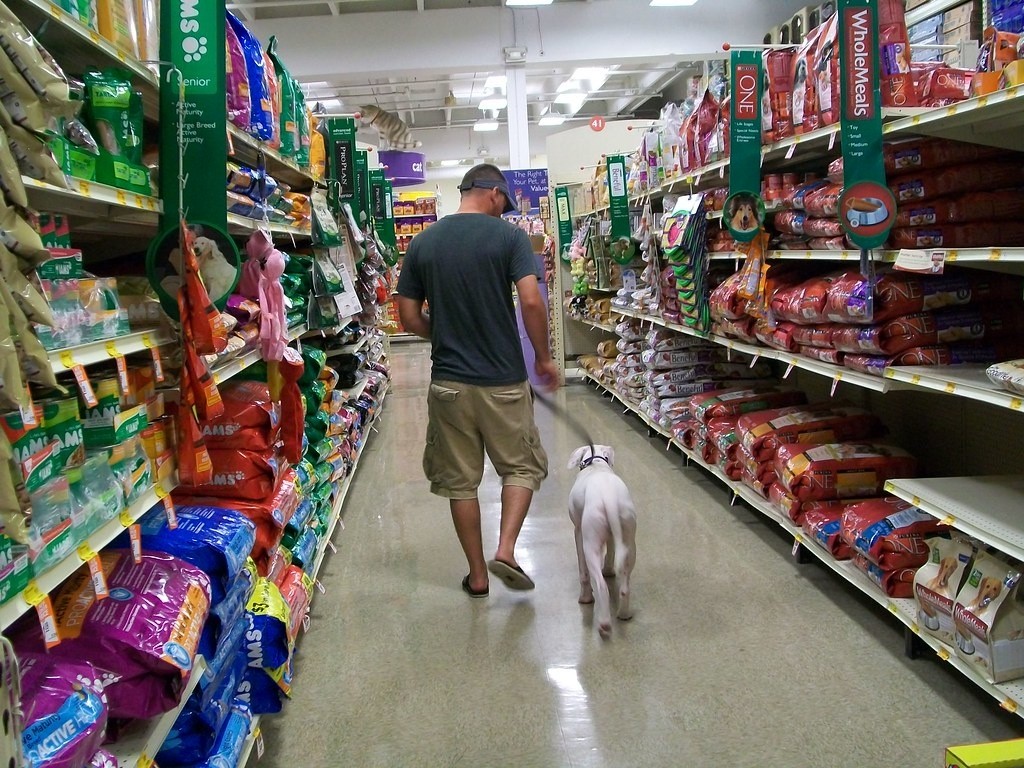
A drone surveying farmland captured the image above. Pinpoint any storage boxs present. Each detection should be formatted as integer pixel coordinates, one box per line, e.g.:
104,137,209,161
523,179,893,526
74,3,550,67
764,0,1024,67
140,391,166,423
81,403,148,447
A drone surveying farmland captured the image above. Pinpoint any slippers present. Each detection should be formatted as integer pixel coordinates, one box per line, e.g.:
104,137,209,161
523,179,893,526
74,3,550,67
462,573,489,598
488,560,534,590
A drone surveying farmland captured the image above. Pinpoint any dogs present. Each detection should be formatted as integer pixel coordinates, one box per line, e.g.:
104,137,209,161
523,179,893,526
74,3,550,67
193,236,237,302
567,444,637,641
730,196,759,232
919,556,1002,654
612,237,631,260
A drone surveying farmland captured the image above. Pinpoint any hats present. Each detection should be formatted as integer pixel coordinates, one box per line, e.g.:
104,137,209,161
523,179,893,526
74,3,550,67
458,181,519,213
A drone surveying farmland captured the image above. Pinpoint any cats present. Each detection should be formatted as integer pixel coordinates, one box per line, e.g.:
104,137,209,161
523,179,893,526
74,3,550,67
359,104,423,149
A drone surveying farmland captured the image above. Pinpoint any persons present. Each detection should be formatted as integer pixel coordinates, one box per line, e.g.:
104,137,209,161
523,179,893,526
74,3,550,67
395,164,560,598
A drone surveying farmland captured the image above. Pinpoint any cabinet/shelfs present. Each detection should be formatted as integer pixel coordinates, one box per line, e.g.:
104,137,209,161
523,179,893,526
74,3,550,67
520,45,1024,722
378,213,438,343
1,0,396,768
